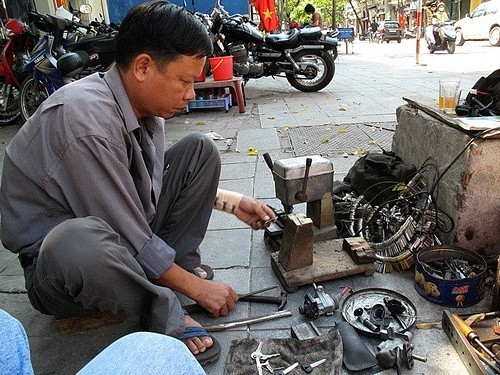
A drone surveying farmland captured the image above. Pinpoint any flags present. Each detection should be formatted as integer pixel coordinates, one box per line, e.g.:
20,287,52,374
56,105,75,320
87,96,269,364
252,0,280,32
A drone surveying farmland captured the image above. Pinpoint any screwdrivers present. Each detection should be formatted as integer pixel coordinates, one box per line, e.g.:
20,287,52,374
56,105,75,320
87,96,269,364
451,313,500,366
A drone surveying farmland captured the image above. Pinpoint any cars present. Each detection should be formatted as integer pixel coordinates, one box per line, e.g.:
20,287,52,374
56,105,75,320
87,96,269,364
404,26,424,39
453,0,500,46
376,21,402,43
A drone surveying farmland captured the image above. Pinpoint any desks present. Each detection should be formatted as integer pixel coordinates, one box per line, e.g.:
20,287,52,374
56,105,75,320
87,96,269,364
193,75,246,113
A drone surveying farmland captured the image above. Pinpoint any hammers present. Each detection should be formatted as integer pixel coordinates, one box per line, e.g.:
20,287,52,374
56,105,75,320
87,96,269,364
237,291,288,310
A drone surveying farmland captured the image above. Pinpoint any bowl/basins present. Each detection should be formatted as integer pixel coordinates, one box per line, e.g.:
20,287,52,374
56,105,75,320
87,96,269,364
414,245,487,308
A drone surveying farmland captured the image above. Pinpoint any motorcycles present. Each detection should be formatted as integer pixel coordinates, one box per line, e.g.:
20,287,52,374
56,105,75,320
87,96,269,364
176,0,339,93
424,17,457,54
0,4,123,124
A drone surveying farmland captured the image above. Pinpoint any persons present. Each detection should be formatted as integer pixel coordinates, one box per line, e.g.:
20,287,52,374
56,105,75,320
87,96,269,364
0,309,207,375
428,2,448,46
371,20,379,32
0,0,276,364
305,4,322,28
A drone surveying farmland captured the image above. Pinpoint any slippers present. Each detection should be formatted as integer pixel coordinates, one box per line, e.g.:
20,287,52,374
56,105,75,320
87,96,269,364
176,308,222,361
186,264,215,280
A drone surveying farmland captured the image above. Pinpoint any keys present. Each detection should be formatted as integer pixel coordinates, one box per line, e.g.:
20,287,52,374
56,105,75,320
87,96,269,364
252,342,264,359
260,353,280,360
301,359,326,373
260,359,273,373
274,362,298,375
265,216,278,224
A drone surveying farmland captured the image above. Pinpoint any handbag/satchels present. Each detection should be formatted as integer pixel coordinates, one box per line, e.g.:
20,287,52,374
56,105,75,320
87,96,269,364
343,149,418,206
466,68,500,115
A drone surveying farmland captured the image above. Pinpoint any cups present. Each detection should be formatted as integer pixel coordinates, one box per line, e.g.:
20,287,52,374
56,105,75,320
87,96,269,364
439,80,460,109
445,88,461,114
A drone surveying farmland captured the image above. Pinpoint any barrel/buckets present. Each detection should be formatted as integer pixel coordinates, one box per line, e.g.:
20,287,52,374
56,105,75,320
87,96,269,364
208,56,234,81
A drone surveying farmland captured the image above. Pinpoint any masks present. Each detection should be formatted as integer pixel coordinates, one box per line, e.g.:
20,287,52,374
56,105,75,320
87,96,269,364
439,7,444,11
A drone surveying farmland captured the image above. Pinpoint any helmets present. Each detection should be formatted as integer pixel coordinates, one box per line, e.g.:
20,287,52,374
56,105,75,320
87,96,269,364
437,3,446,8
57,52,82,77
431,3,437,7
